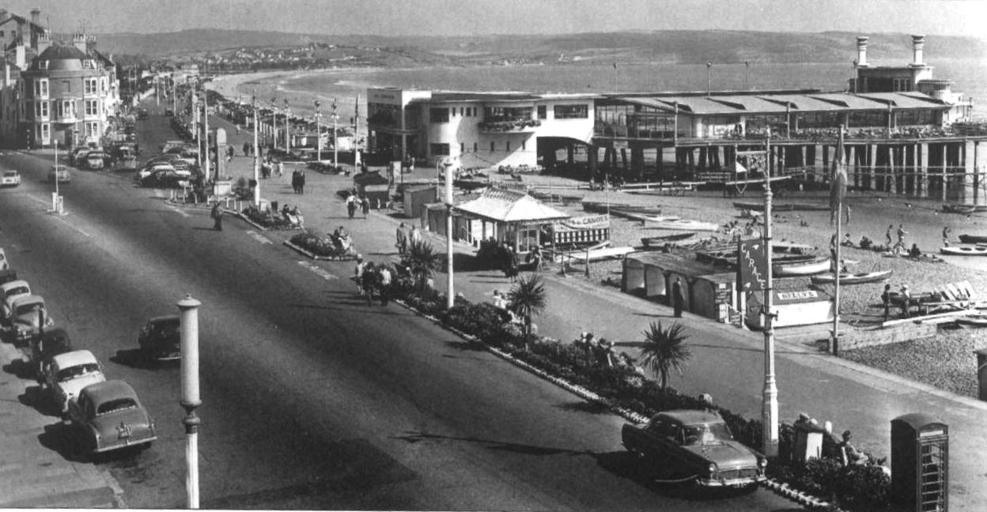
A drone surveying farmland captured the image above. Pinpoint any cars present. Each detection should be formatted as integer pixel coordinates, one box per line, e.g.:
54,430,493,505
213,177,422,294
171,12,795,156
69,103,213,188
621,407,768,497
138,312,180,369
0,168,22,187
0,245,156,464
48,164,73,185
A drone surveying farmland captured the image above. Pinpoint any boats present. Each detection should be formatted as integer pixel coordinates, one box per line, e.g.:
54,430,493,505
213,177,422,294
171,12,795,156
810,262,894,284
840,281,986,327
939,243,986,256
641,231,699,249
958,234,987,242
770,240,832,277
527,189,719,232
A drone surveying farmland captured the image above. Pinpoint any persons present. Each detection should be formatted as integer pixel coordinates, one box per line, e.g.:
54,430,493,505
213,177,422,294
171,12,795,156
491,289,538,334
719,202,952,327
838,429,888,467
671,276,685,320
209,137,434,310
580,330,645,384
532,245,544,272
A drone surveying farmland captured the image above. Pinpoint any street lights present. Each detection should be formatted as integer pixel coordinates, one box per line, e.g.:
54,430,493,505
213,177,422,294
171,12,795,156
729,122,784,465
51,138,61,216
251,87,344,208
